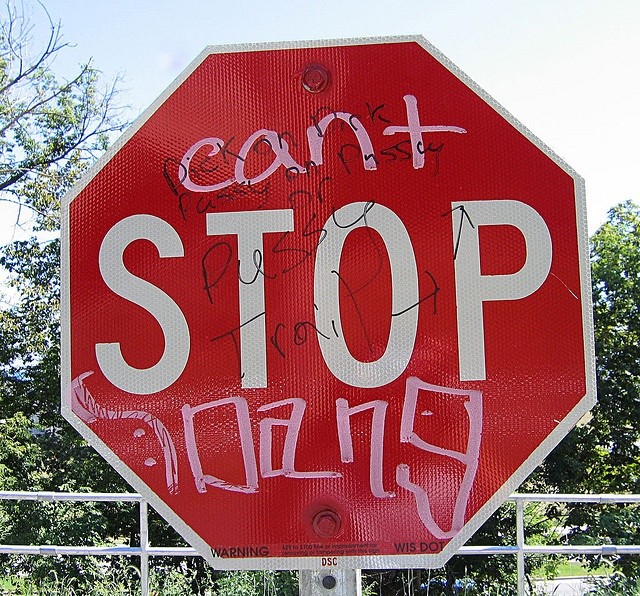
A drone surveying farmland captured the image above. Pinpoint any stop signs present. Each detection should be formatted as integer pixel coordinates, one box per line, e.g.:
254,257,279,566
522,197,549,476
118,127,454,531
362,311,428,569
58,34,598,573
